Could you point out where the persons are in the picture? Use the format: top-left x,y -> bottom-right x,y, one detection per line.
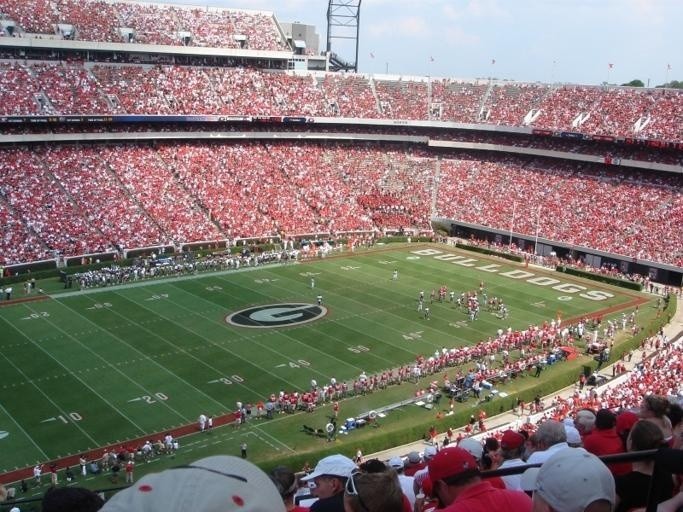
2,49 -> 682,275
2,0 -> 292,53
1,389 -> 300,512
393,269 -> 397,279
5,273 -> 154,299
299,276 -> 683,512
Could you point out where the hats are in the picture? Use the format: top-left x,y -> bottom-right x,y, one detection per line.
103,396 -> 682,510
